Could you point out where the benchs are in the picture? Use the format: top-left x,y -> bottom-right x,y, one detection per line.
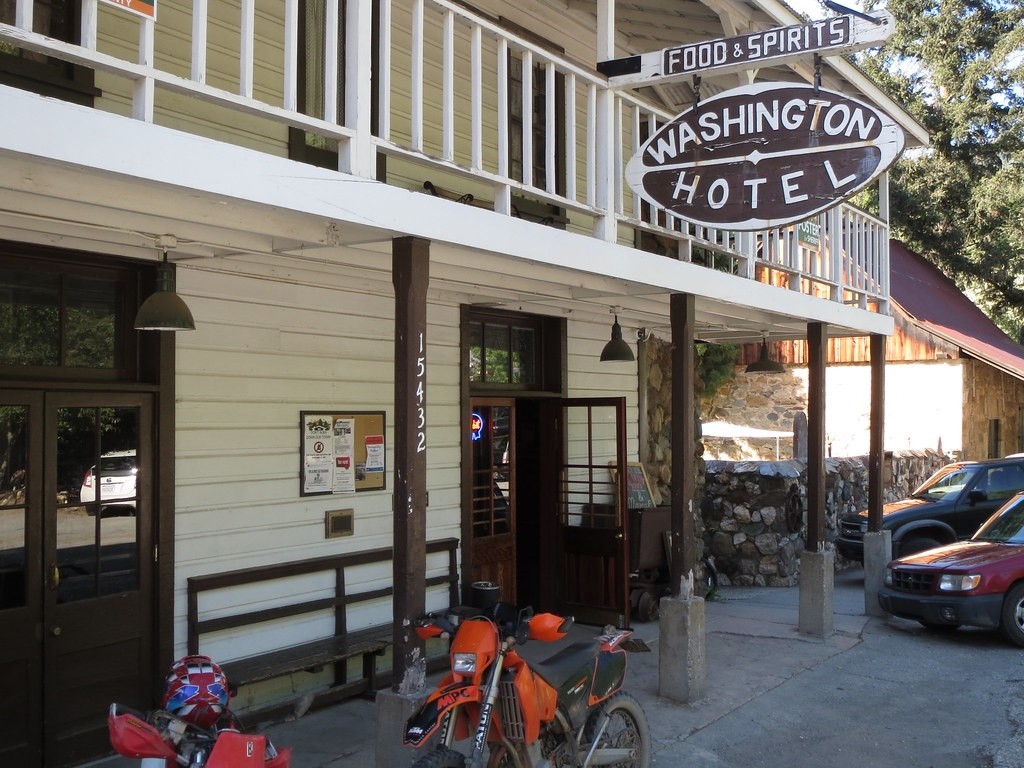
187,536 -> 481,732
423,181 -> 554,226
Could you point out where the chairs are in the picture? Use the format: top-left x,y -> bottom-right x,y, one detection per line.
986,471 -> 1024,499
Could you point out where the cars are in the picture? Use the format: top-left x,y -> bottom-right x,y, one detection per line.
79,449 -> 137,515
876,489 -> 1024,645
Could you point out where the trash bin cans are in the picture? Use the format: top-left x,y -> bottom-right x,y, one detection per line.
471,580 -> 500,609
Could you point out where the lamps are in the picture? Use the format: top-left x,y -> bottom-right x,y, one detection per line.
134,234 -> 196,330
600,306 -> 635,363
745,331 -> 786,373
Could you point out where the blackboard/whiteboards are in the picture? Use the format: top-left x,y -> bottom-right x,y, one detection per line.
608,461 -> 656,508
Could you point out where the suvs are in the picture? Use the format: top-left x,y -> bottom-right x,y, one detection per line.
833,453 -> 1024,574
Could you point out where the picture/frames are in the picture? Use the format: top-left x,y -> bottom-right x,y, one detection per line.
607,460 -> 656,509
299,410 -> 387,498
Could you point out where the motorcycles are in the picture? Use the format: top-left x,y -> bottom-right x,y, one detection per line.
104,653 -> 292,768
402,602 -> 652,768
